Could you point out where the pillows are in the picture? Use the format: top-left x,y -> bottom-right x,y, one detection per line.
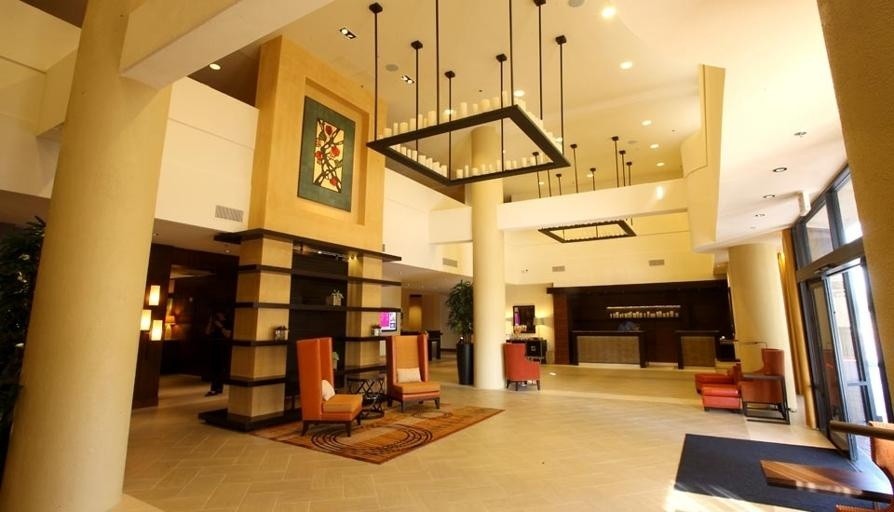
397,368 -> 422,383
321,380 -> 335,401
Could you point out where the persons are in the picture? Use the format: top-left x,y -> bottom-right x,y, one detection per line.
204,305 -> 233,397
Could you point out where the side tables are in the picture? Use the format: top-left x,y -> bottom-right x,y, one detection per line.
347,374 -> 385,419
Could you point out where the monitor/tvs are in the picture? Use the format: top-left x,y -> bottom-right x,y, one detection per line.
380,312 -> 397,332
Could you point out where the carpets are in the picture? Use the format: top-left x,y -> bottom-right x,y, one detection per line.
675,433 -> 890,512
248,386 -> 505,465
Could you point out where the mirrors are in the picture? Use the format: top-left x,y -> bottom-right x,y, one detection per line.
512,306 -> 536,333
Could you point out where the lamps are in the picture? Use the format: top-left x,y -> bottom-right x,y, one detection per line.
532,136 -> 636,243
140,284 -> 163,343
366,0 -> 571,187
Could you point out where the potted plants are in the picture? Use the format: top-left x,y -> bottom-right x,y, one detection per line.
445,280 -> 473,385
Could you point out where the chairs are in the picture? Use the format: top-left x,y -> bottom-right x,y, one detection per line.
386,334 -> 440,413
296,337 -> 362,436
503,344 -> 540,391
738,348 -> 790,425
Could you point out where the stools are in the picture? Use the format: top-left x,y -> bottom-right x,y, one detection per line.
695,373 -> 734,393
701,383 -> 743,413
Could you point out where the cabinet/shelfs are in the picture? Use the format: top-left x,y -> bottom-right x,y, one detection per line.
200,228 -> 401,432
507,339 -> 547,364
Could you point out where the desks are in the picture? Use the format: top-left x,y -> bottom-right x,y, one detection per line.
402,330 -> 443,361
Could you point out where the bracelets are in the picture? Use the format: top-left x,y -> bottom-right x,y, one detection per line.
220,326 -> 224,329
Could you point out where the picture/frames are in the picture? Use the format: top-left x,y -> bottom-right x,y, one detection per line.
298,96 -> 355,213
